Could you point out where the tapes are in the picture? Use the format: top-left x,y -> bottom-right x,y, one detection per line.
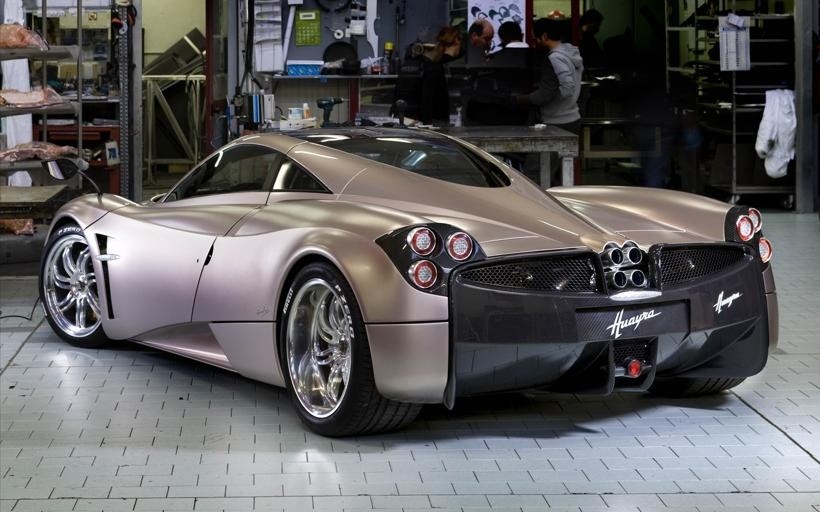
334,29 -> 344,40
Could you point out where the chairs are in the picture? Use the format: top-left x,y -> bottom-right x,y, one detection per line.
272,162 -> 327,190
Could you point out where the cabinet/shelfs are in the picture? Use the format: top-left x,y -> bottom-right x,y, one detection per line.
1,0 -> 83,276
664,0 -> 815,214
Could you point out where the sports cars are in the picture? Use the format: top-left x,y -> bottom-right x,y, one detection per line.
39,124 -> 781,438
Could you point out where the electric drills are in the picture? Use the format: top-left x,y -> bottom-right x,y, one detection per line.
316,96 -> 350,128
392,99 -> 408,129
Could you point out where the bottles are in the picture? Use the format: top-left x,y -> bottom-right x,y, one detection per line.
382,42 -> 398,76
302,103 -> 311,119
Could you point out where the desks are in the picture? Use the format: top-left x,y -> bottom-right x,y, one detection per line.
32,123 -> 122,196
441,124 -> 579,190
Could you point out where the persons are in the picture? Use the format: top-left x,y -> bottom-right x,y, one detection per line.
388,8 -> 605,189
